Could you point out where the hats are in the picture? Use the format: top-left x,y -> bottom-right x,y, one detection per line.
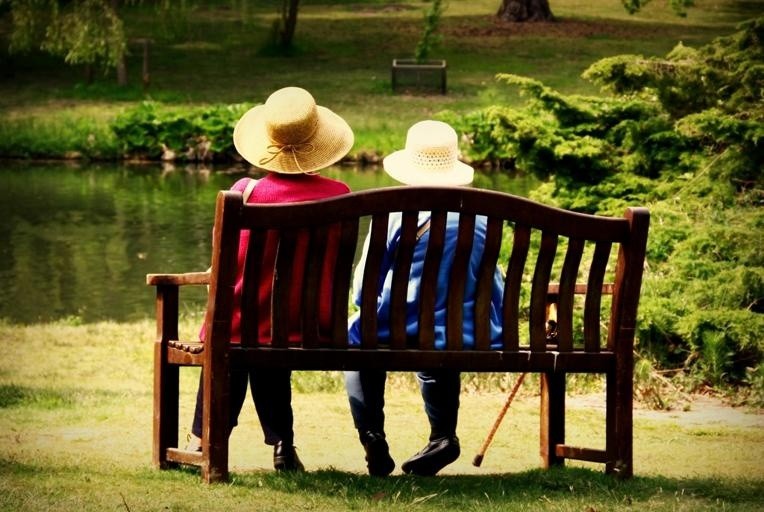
233,86 -> 356,176
382,117 -> 475,188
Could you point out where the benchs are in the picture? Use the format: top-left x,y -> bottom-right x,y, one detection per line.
143,187 -> 652,487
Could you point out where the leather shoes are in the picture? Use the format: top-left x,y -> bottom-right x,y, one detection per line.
401,437 -> 460,477
273,440 -> 305,473
362,431 -> 395,479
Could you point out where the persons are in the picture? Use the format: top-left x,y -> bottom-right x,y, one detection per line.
192,86 -> 358,472
347,119 -> 506,476
